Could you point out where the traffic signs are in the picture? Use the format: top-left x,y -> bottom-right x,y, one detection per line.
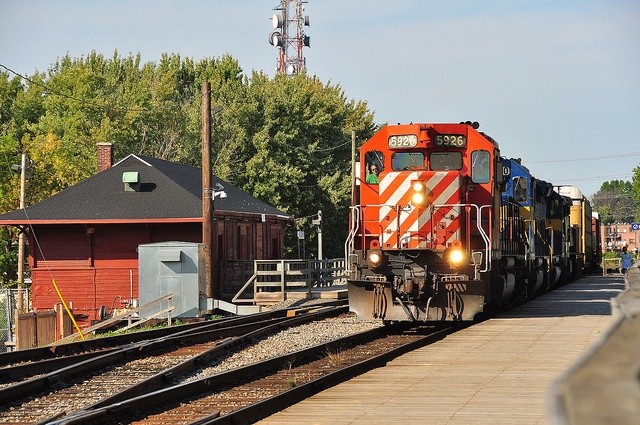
631,222 -> 640,229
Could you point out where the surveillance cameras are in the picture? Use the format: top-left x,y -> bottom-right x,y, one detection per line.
219,191 -> 227,200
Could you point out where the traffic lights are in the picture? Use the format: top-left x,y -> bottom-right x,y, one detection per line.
303,16 -> 310,48
268,11 -> 284,47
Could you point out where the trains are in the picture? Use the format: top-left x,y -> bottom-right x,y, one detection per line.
346,122 -> 602,324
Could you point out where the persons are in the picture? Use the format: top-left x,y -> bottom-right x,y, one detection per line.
620,247 -> 633,274
366,163 -> 380,184
